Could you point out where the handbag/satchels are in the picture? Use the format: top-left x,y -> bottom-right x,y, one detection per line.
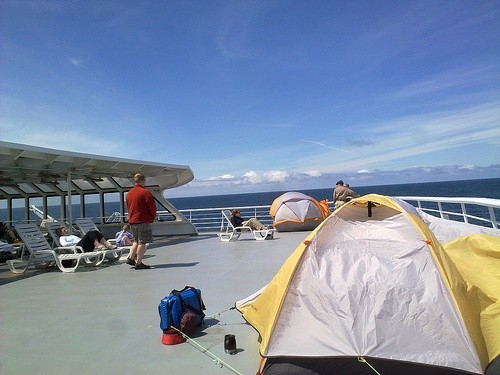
158,286 -> 206,329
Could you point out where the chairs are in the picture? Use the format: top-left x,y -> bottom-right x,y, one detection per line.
5,217 -> 149,273
218,210 -> 275,242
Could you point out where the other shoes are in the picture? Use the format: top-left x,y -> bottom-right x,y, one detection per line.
107,245 -> 117,249
125,257 -> 136,265
135,262 -> 150,269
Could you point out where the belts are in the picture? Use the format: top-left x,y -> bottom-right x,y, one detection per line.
337,200 -> 344,201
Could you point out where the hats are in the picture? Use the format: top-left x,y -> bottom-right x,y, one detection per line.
336,181 -> 343,185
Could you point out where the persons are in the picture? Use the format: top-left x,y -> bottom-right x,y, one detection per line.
56,226 -> 117,253
231,210 -> 267,232
125,173 -> 157,270
332,180 -> 358,209
115,225 -> 133,247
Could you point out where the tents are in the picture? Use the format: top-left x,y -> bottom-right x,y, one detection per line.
233,193 -> 500,375
269,192 -> 331,232
